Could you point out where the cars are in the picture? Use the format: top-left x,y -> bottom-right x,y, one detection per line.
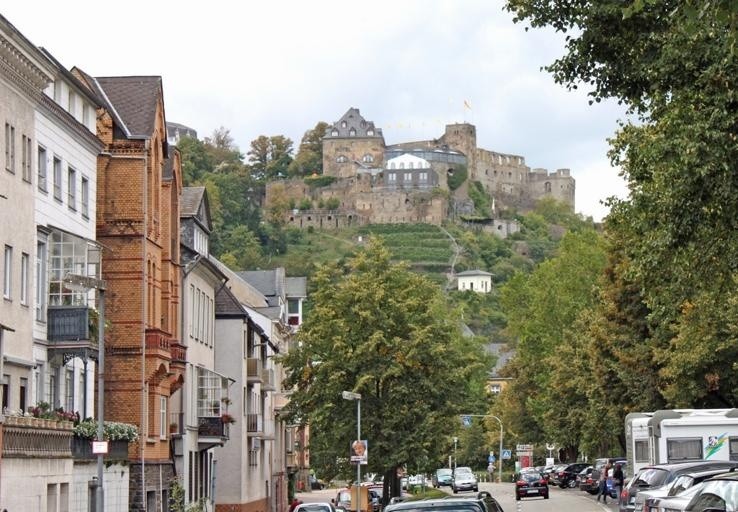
285,466 -> 503,512
511,458 -> 738,512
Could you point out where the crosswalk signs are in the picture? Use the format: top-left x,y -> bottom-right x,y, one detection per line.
501,450 -> 511,460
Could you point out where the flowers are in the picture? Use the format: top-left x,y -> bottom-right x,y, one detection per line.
73,421 -> 139,443
35,401 -> 80,420
88,308 -> 110,330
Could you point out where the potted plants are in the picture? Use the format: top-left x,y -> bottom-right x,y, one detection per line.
222,414 -> 237,425
170,423 -> 177,433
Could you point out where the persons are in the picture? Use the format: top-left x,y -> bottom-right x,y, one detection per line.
289,498 -> 299,512
597,459 -> 624,504
487,462 -> 497,482
351,440 -> 367,461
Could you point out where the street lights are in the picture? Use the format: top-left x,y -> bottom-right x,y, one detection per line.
341,390 -> 363,512
452,435 -> 458,469
61,271 -> 107,511
